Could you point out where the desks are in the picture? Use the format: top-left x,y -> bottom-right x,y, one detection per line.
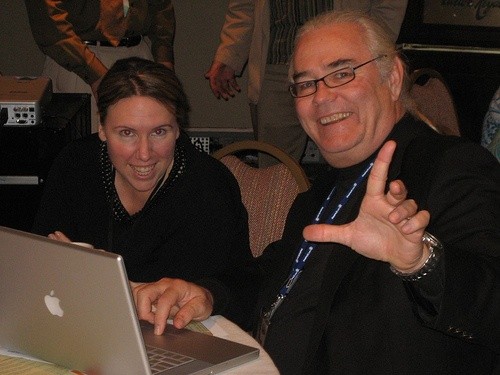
0,283 -> 279,375
0,94 -> 94,176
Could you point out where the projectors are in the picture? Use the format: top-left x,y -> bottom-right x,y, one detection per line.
0,73 -> 54,128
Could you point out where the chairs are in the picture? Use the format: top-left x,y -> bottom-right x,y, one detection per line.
210,139 -> 311,257
409,68 -> 461,138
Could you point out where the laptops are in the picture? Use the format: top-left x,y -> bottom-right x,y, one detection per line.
0,227 -> 259,375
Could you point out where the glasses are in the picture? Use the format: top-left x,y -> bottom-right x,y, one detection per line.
287,54 -> 389,99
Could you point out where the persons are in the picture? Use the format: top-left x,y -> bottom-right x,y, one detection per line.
132,8 -> 500,375
26,0 -> 176,135
31,57 -> 254,283
202,0 -> 408,169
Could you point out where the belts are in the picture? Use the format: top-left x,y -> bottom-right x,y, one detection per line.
82,33 -> 141,48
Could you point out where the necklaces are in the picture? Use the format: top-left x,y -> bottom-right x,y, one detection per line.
99,140 -> 186,224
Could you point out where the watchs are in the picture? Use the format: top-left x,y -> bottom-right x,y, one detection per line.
389,231 -> 445,282
